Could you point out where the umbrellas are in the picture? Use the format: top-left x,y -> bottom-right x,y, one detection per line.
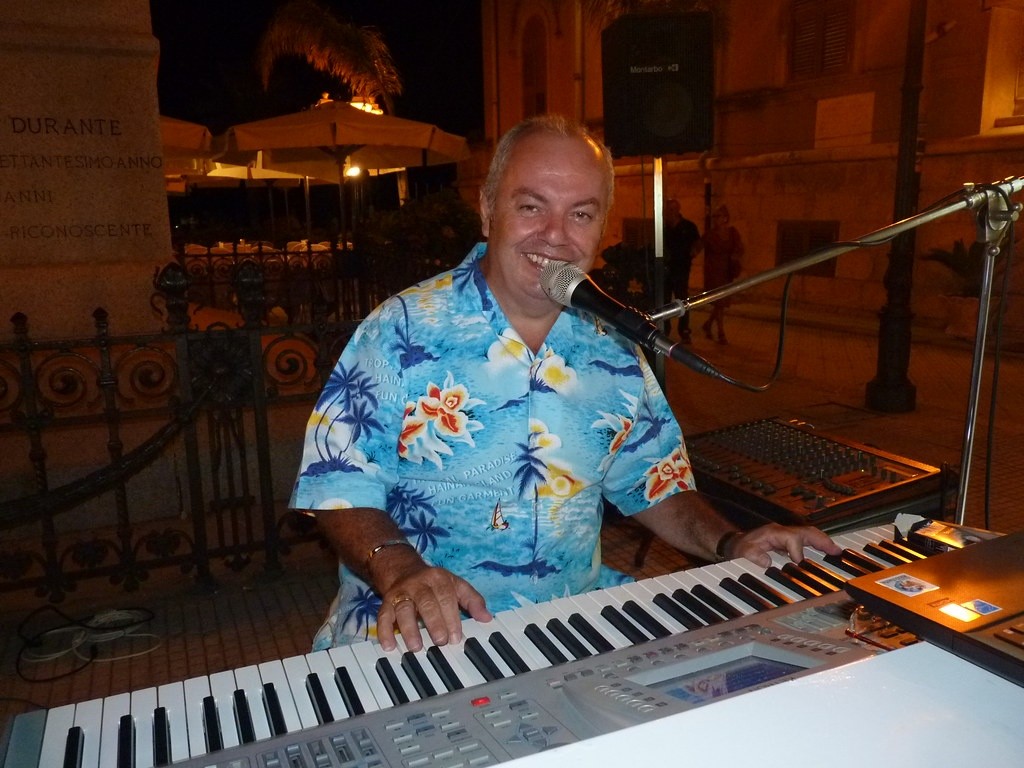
161,100 -> 468,249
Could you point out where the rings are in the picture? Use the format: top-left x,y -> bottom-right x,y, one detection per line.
392,595 -> 419,617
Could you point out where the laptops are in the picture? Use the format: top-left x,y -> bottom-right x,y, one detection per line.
845,530 -> 1024,688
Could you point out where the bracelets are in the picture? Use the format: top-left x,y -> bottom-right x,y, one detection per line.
363,538 -> 417,583
715,530 -> 746,563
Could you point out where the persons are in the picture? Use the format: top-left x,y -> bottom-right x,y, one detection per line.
653,200 -> 703,344
702,206 -> 744,345
288,115 -> 843,652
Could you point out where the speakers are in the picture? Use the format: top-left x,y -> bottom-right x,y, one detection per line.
602,10 -> 714,158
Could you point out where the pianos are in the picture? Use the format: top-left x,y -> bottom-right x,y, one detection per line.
1,512 -> 1024,768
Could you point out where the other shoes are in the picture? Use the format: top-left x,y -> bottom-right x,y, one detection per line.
718,333 -> 728,344
703,322 -> 713,339
680,329 -> 692,343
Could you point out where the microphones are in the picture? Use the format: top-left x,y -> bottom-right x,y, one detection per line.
539,258 -> 721,377
